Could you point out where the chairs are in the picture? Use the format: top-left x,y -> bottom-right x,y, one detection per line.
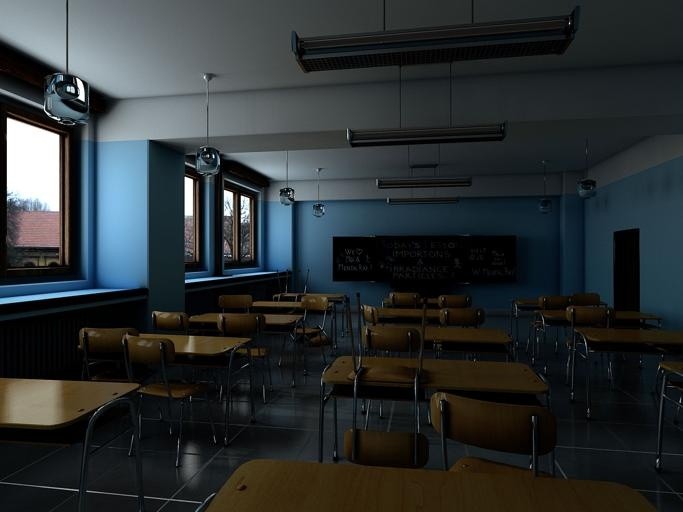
277,269 -> 310,313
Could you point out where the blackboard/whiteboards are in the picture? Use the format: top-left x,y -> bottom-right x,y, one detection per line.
331,233 -> 521,285
